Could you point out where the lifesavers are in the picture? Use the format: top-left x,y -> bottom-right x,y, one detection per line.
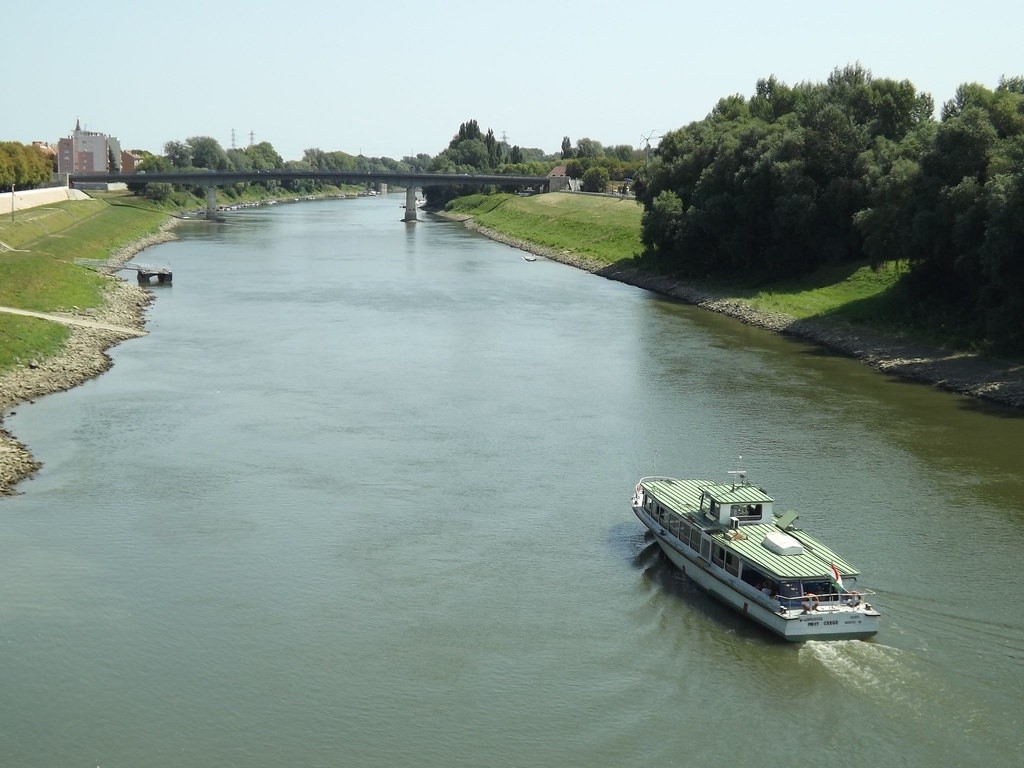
846,590 -> 862,607
801,593 -> 818,611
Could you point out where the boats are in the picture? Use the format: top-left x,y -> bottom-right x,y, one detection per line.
629,456 -> 883,640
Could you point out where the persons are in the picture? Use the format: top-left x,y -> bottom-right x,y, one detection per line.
754,580 -> 772,596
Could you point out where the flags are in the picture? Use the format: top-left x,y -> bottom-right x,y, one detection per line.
831,562 -> 843,587
